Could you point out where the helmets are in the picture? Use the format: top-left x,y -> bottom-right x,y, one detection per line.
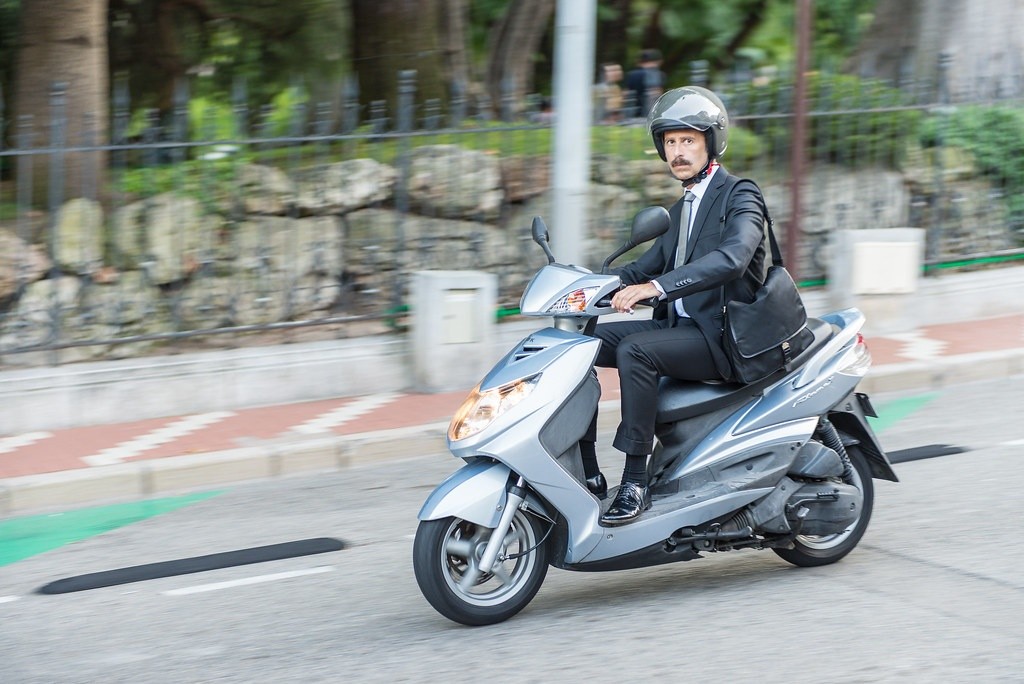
645,86 -> 729,163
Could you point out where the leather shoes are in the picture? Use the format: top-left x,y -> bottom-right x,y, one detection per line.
602,481 -> 653,526
586,474 -> 607,500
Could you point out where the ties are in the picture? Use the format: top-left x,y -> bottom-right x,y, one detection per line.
667,192 -> 696,326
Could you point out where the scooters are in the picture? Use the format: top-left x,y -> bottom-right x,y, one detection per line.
411,203 -> 901,627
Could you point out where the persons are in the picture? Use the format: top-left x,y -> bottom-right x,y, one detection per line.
580,86 -> 767,523
530,46 -> 777,129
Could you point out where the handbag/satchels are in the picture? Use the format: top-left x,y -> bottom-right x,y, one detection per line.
722,266 -> 816,386
